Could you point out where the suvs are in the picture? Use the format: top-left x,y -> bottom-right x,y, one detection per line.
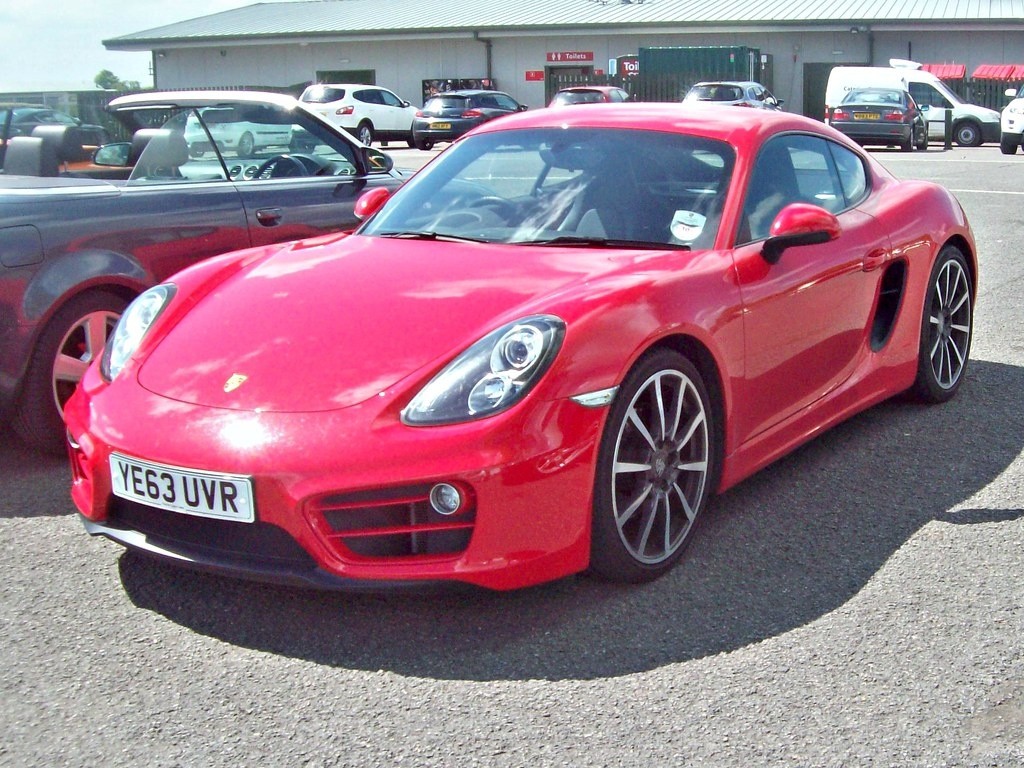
291,83 -> 421,154
184,106 -> 291,158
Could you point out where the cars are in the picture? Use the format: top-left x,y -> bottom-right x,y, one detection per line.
681,80 -> 784,111
1000,82 -> 1024,155
540,85 -> 636,143
0,108 -> 182,178
412,89 -> 527,150
830,85 -> 929,153
64,102 -> 979,590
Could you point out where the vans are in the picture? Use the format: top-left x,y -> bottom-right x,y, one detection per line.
825,59 -> 1002,146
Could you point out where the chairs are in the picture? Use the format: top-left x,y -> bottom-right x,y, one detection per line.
557,166 -> 640,241
691,181 -> 784,244
31,125 -> 89,178
130,129 -> 189,180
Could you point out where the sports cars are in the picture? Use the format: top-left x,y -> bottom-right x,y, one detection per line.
0,92 -> 493,459
